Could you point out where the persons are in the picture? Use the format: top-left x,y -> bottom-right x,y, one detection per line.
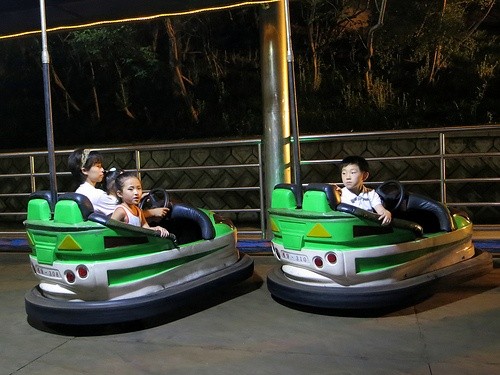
68,148 -> 169,237
333,155 -> 392,225
